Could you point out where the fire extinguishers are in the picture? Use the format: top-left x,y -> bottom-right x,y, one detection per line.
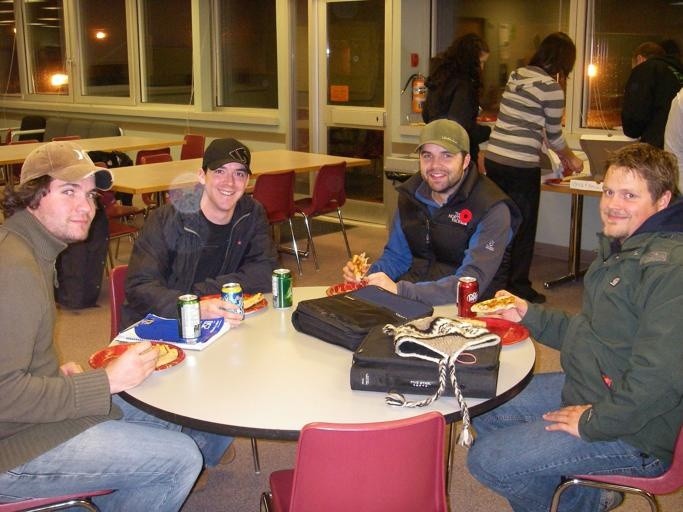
401,73 -> 427,113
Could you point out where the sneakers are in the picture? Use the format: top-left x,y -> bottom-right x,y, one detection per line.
507,284 -> 546,304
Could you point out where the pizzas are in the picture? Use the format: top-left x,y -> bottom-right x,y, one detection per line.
244,293 -> 264,309
352,252 -> 371,281
143,343 -> 169,356
471,295 -> 516,313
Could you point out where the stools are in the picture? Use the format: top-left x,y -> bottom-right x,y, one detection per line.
0,479 -> 114,512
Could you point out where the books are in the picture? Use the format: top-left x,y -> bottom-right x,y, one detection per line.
115,314 -> 230,351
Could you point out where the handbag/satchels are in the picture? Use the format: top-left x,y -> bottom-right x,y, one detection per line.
351,324 -> 501,398
291,285 -> 433,351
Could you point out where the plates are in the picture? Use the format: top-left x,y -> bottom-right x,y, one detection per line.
470,316 -> 530,346
326,279 -> 366,296
199,293 -> 268,316
87,340 -> 186,374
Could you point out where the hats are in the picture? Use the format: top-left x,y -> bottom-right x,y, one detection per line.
202,139 -> 252,176
414,119 -> 471,156
20,140 -> 114,191
382,315 -> 501,448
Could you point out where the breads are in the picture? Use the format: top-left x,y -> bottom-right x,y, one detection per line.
156,348 -> 179,368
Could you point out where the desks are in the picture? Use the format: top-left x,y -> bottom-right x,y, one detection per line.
540,167 -> 609,291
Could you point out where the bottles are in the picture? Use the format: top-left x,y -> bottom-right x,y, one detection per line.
177,294 -> 201,339
221,282 -> 245,324
271,268 -> 293,310
456,277 -> 480,319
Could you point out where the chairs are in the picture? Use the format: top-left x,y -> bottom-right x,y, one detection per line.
179,133 -> 206,160
40,118 -> 123,144
253,171 -> 302,276
3,116 -> 44,145
109,265 -> 128,349
550,416 -> 683,511
94,162 -> 135,229
141,154 -> 179,221
104,220 -> 137,275
262,413 -> 447,512
293,161 -> 351,265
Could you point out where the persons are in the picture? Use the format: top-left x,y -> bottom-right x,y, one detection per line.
484,31 -> 583,303
113,137 -> 279,491
422,33 -> 490,176
343,120 -> 521,308
662,85 -> 683,197
467,142 -> 682,511
0,142 -> 236,512
620,40 -> 683,148
